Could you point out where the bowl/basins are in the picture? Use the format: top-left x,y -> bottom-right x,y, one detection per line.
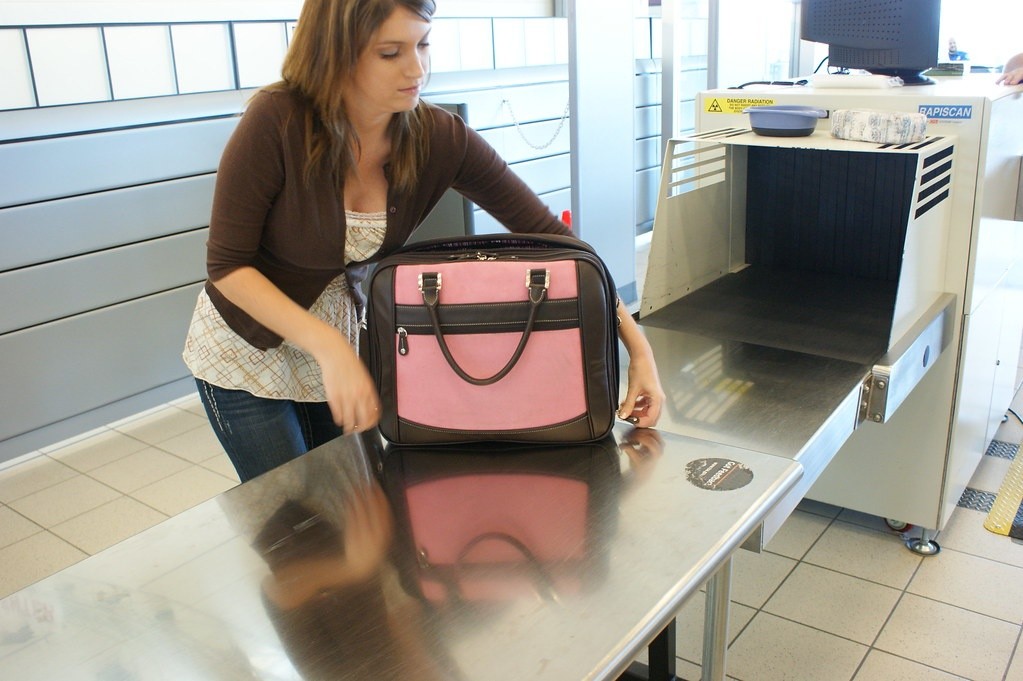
741,105 -> 827,137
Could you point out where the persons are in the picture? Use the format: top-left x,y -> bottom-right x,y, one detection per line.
220,427 -> 665,680
182,2 -> 667,482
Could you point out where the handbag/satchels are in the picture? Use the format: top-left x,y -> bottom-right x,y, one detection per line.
376,444 -> 617,621
365,231 -> 621,448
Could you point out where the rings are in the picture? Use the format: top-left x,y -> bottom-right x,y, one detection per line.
373,406 -> 383,411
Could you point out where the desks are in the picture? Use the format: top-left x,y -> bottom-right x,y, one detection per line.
0,426 -> 806,680
